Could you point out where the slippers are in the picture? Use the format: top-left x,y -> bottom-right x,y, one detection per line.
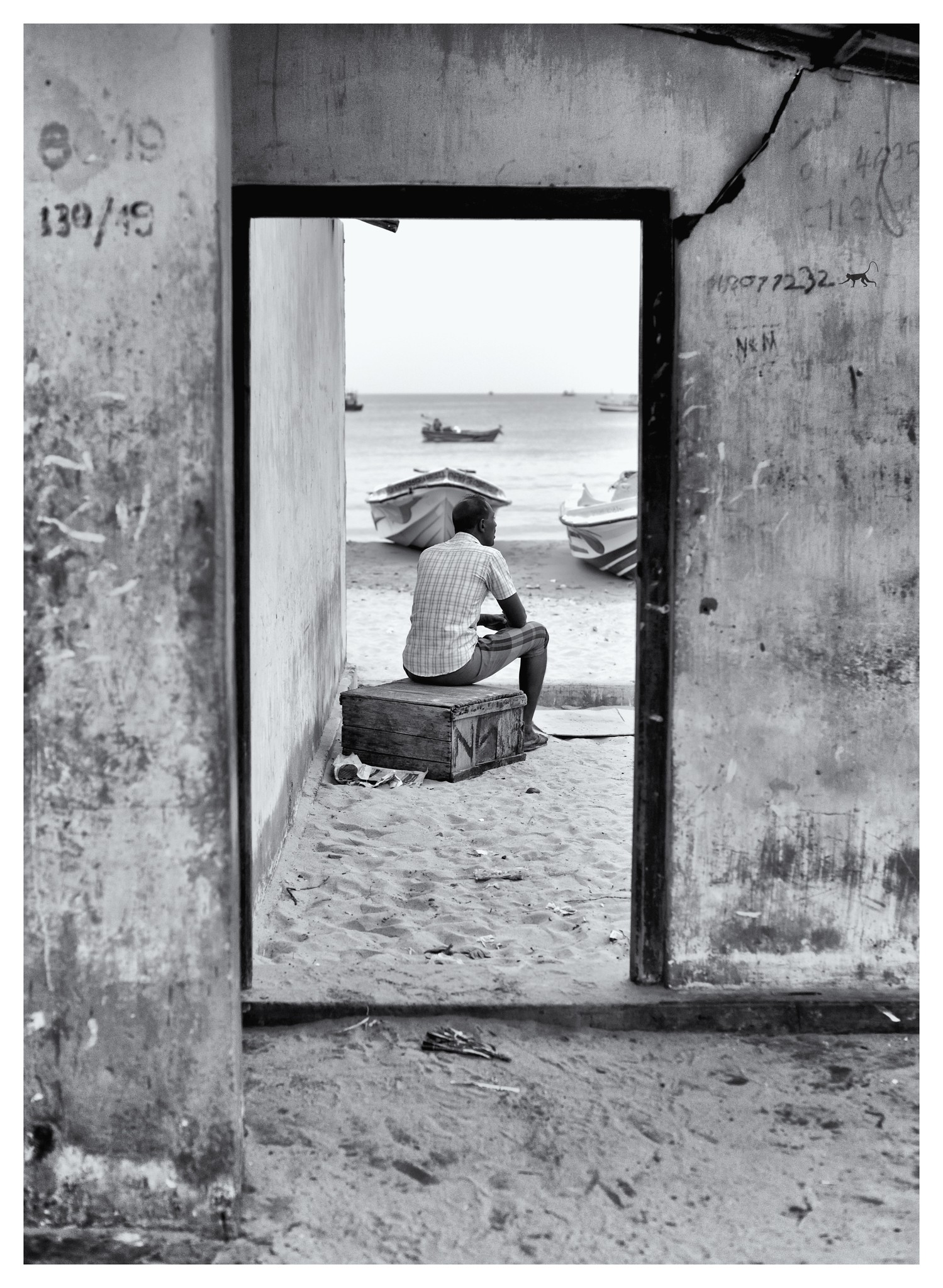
524,733 -> 548,751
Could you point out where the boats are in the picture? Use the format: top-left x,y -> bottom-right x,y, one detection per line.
557,473 -> 637,584
364,466 -> 511,555
422,418 -> 502,441
345,392 -> 363,411
595,399 -> 639,413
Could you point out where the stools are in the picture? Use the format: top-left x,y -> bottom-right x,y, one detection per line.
339,679 -> 527,783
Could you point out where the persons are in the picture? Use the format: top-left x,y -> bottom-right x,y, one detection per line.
402,493 -> 550,752
